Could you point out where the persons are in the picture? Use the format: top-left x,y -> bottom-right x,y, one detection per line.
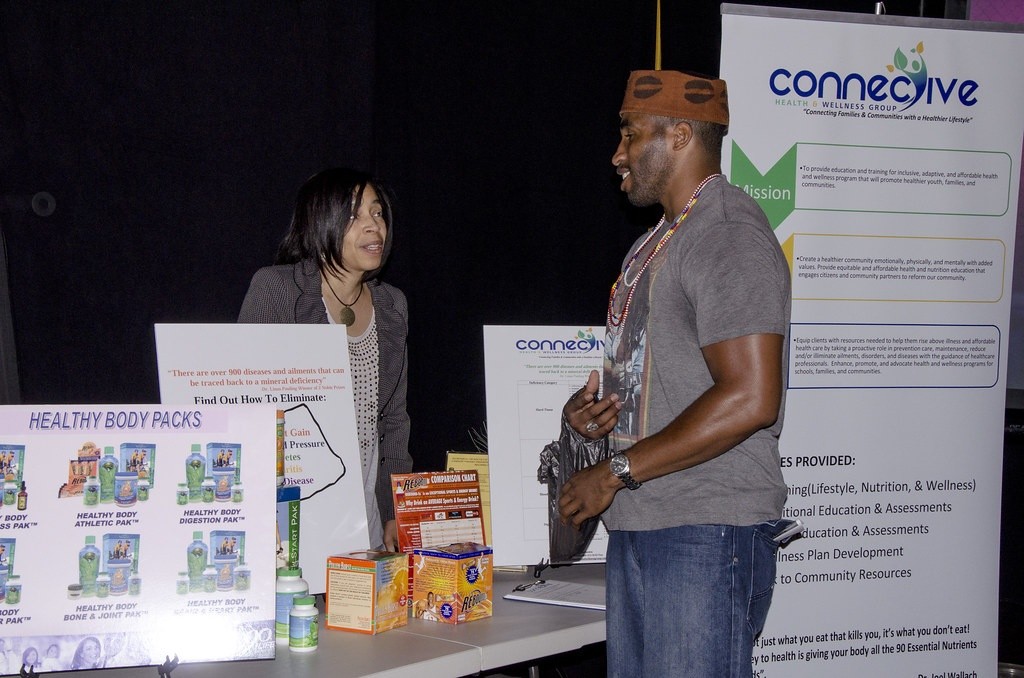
557,68 -> 793,675
234,166 -> 413,553
4,451 -> 16,475
136,450 -> 146,472
226,537 -> 238,555
220,537 -> 229,555
0,451 -> 6,474
113,541 -> 123,558
223,450 -> 233,468
416,592 -> 445,623
217,449 -> 225,468
130,449 -> 139,471
120,541 -> 132,560
0,544 -> 6,566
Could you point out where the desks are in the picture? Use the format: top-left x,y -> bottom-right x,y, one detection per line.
0,562 -> 610,678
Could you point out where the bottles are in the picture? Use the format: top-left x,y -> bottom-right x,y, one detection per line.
83,475 -> 100,505
276,410 -> 286,486
5,575 -> 21,604
3,479 -> 18,505
275,566 -> 309,646
0,473 -> 5,507
289,595 -> 319,652
0,565 -> 8,603
137,477 -> 150,501
175,531 -> 251,595
98,446 -> 119,504
67,535 -> 142,598
114,472 -> 138,506
18,481 -> 28,510
230,482 -> 244,503
212,467 -> 235,502
176,483 -> 189,505
201,476 -> 216,502
185,443 -> 206,503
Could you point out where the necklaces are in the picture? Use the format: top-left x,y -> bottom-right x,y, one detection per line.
607,172 -> 727,338
321,267 -> 364,327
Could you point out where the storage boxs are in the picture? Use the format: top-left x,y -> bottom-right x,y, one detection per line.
413,541 -> 493,624
0,538 -> 16,575
210,530 -> 245,566
0,444 -> 25,491
276,484 -> 301,567
120,442 -> 156,488
325,549 -> 408,636
206,442 -> 241,485
103,533 -> 140,573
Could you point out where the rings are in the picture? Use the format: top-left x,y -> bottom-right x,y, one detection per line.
587,420 -> 599,433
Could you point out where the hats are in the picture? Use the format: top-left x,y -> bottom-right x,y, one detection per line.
618,70 -> 730,126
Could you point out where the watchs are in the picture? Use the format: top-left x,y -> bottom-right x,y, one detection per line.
610,448 -> 643,491
